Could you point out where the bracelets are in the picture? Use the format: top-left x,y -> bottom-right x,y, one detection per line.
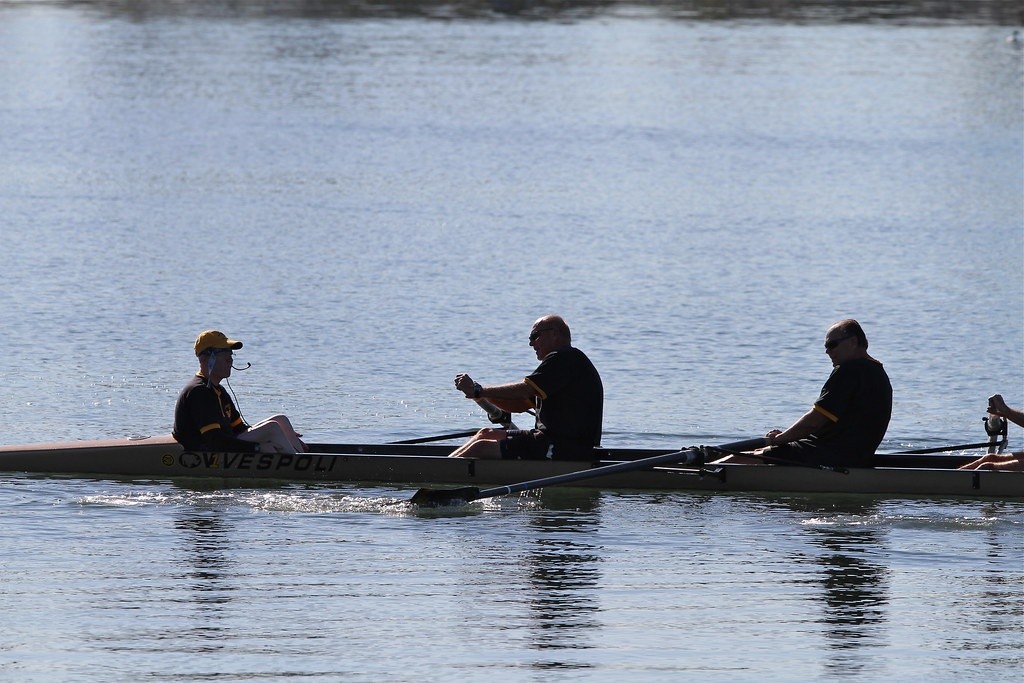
255,443 -> 260,452
999,405 -> 1007,411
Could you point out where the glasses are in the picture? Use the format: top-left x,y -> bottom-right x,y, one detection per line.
825,335 -> 855,350
529,328 -> 555,340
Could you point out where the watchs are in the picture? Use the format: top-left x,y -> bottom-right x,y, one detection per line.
473,383 -> 482,397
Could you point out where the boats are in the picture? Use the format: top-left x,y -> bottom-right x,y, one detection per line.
1,435 -> 1024,502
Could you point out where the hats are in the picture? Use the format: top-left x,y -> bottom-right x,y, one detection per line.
195,330 -> 242,357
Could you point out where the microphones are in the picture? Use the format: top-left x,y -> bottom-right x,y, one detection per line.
232,362 -> 251,370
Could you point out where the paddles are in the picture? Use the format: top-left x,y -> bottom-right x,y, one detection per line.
984,409 -> 1005,452
408,437 -> 768,510
466,394 -> 520,433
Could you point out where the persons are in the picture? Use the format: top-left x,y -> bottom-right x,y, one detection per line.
958,395 -> 1024,470
449,315 -> 605,460
173,330 -> 304,453
710,320 -> 893,469
